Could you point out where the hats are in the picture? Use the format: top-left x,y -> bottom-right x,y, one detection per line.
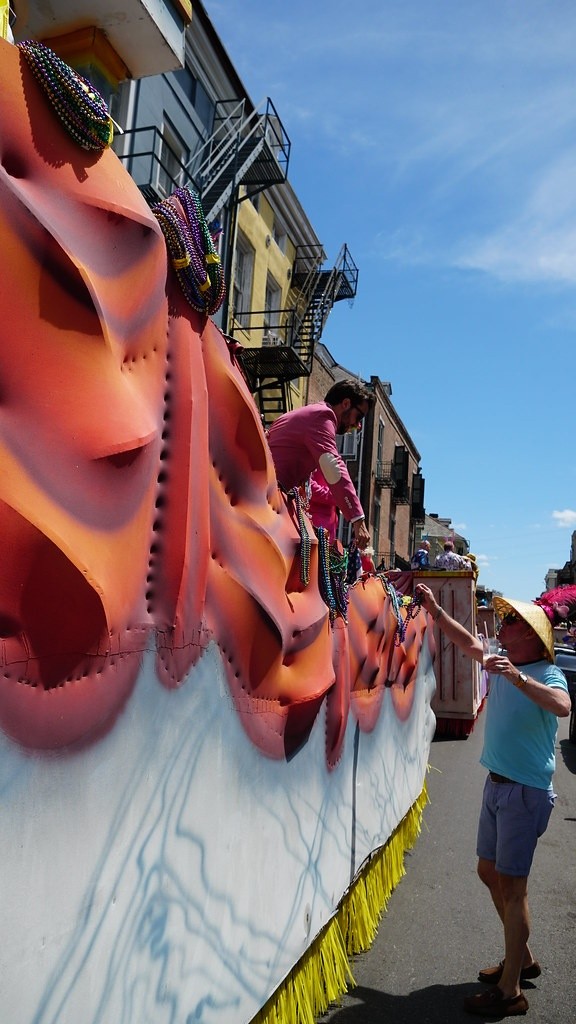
492,584 -> 576,664
462,553 -> 476,565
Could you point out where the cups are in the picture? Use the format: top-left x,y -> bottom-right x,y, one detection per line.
482,638 -> 499,670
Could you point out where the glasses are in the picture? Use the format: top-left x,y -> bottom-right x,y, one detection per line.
353,405 -> 364,422
502,614 -> 528,625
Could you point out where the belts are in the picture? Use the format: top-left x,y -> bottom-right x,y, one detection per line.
489,771 -> 517,784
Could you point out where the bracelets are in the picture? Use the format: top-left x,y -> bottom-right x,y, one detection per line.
434,606 -> 443,623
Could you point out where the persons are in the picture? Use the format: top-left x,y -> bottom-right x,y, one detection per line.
266,377 -> 378,551
411,540 -> 431,565
433,541 -> 471,572
415,583 -> 572,1016
306,467 -> 340,543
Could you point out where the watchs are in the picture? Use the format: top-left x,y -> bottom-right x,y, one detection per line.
513,671 -> 528,688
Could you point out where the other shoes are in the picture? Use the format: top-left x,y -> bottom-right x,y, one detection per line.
463,989 -> 530,1014
479,959 -> 541,979
